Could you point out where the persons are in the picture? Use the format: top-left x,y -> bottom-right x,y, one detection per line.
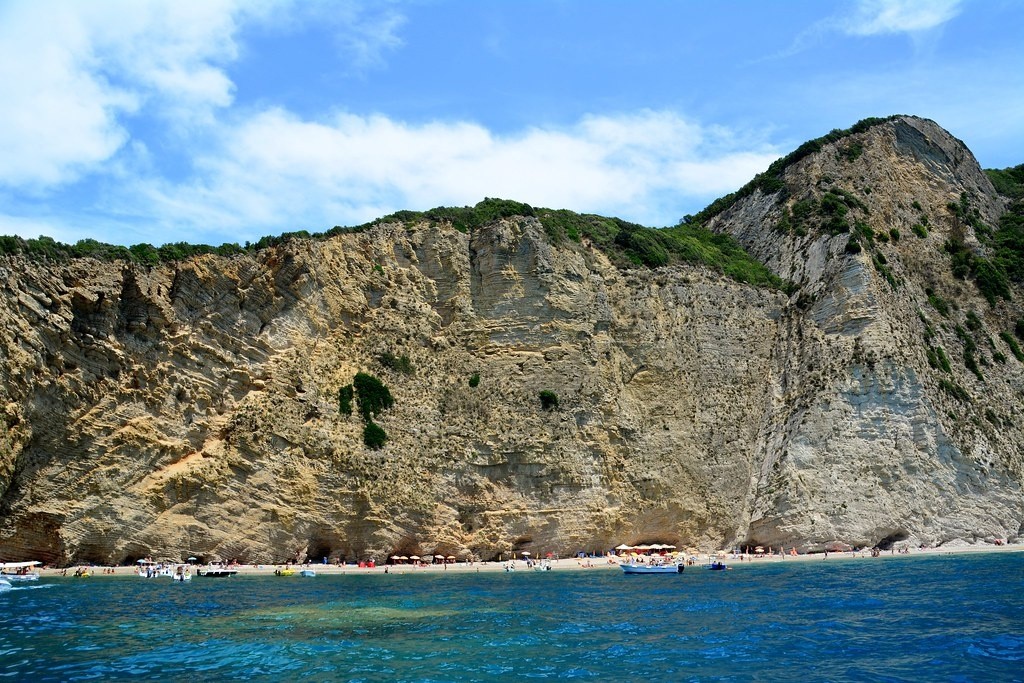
323,556 -> 380,568
0,562 -> 119,577
466,555 -> 750,568
769,541 -> 930,560
993,538 -> 1009,544
136,559 -> 242,573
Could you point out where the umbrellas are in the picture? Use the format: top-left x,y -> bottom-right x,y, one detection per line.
138,559 -> 146,568
188,557 -> 197,564
391,555 -> 455,564
755,545 -> 763,555
615,542 -> 677,555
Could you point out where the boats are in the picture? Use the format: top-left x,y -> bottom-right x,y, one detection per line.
301,570 -> 316,577
196,559 -> 238,577
172,564 -> 193,581
709,554 -> 726,571
614,543 -> 679,573
1,560 -> 42,581
139,558 -> 158,579
274,570 -> 295,578
